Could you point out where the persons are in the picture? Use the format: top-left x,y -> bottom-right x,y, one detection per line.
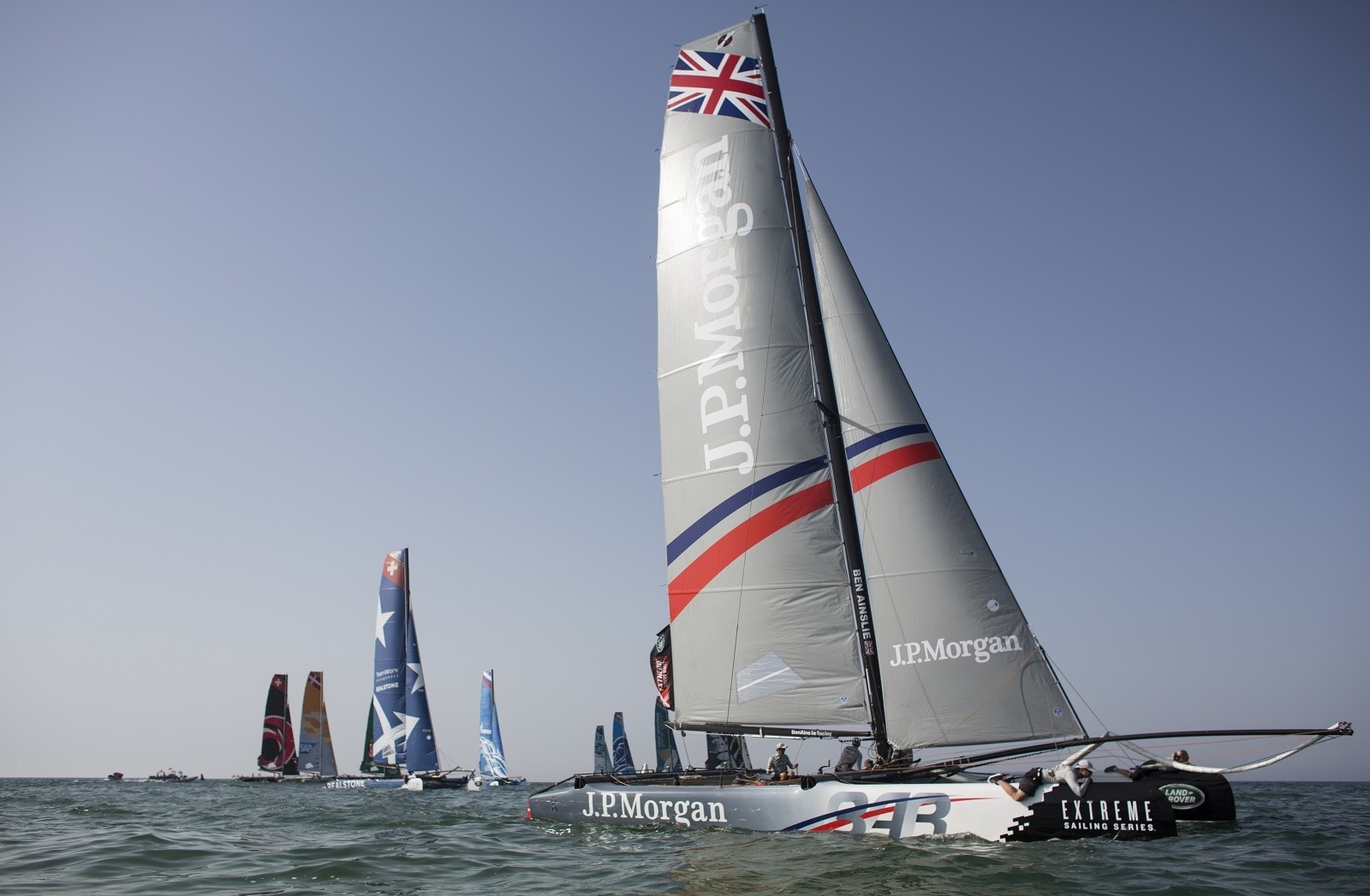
1104,749 -> 1190,779
835,738 -> 904,772
766,743 -> 798,781
987,760 -> 1097,802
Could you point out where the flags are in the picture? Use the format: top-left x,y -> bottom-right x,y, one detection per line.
650,624 -> 675,711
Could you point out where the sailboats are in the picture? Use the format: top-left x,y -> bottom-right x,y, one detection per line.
521,3 -> 1354,841
479,668 -> 527,786
234,546 -> 483,792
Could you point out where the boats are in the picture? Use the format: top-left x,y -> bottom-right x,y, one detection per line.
147,771 -> 199,783
108,772 -> 124,781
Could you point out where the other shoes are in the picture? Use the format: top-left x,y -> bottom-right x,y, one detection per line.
1103,766 -> 1116,773
988,774 -> 1002,785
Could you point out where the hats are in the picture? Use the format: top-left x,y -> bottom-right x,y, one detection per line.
776,742 -> 789,750
853,738 -> 860,746
1074,760 -> 1095,772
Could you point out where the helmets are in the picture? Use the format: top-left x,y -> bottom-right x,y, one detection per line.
1173,750 -> 1188,764
